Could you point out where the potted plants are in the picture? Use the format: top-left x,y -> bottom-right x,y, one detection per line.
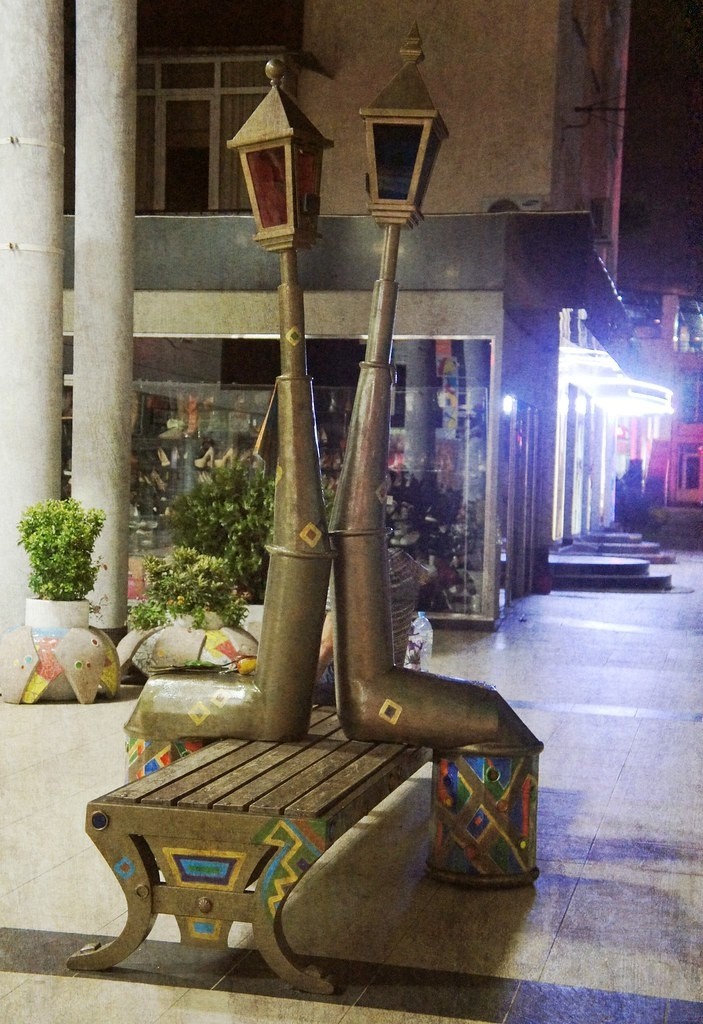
17,498 -> 106,628
123,547 -> 252,633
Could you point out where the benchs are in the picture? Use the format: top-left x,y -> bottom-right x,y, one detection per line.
66,702 -> 432,994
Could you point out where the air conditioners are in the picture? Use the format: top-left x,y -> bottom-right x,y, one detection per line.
482,193 -> 543,213
591,197 -> 613,249
